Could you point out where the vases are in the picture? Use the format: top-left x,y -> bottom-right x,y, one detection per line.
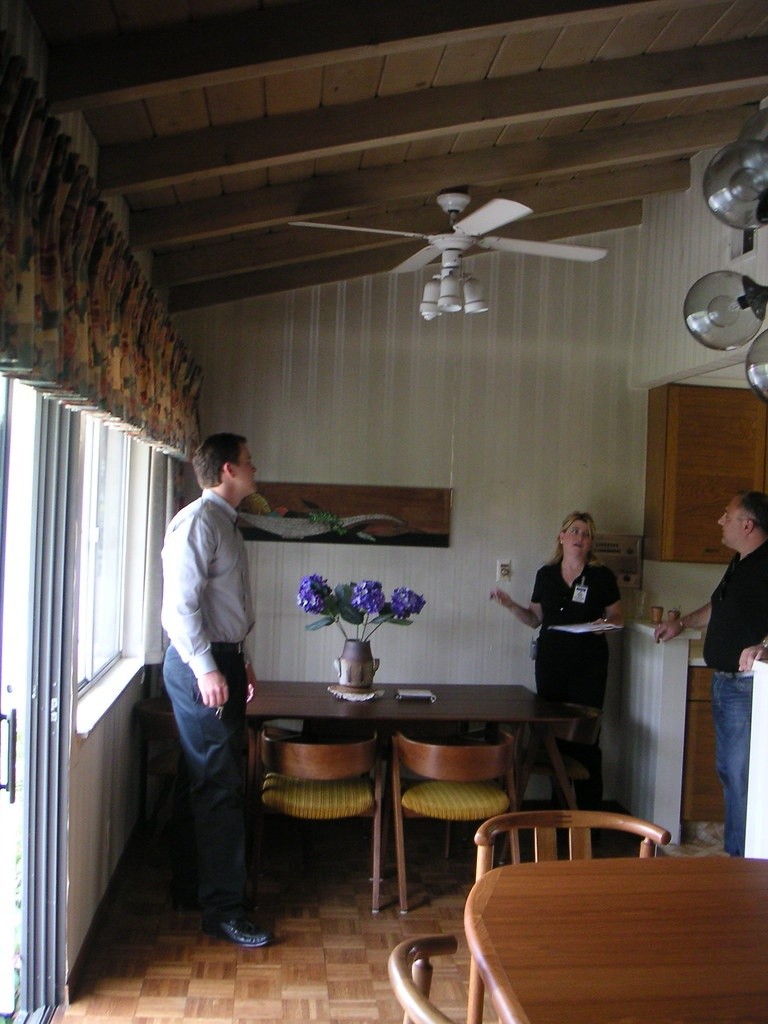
335,639 -> 379,687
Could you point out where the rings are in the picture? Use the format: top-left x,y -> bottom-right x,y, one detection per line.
658,623 -> 660,628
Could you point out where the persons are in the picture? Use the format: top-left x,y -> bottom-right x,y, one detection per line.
489,511 -> 623,858
164,432 -> 275,948
654,490 -> 768,859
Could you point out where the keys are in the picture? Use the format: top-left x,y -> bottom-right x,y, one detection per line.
215,704 -> 223,719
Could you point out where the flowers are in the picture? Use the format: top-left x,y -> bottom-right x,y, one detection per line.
296,575 -> 426,643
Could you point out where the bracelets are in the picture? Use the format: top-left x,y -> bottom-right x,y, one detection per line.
679,617 -> 684,628
760,638 -> 768,647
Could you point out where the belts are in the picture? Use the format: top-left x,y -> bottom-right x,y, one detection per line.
715,672 -> 746,680
211,641 -> 243,655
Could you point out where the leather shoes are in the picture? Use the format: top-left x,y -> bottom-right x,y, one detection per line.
201,909 -> 274,947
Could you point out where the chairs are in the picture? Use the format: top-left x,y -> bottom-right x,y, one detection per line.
388,936 -> 473,1023
133,700 -> 607,917
468,810 -> 672,1024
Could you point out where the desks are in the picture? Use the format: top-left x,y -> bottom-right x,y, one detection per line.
237,679 -> 587,856
463,841 -> 768,1023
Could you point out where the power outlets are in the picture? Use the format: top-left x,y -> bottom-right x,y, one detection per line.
618,573 -> 641,589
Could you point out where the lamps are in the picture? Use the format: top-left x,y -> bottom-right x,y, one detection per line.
418,270 -> 488,319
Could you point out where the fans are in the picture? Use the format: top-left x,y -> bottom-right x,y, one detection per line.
282,191 -> 609,275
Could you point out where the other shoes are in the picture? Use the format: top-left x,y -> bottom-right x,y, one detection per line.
170,887 -> 257,912
